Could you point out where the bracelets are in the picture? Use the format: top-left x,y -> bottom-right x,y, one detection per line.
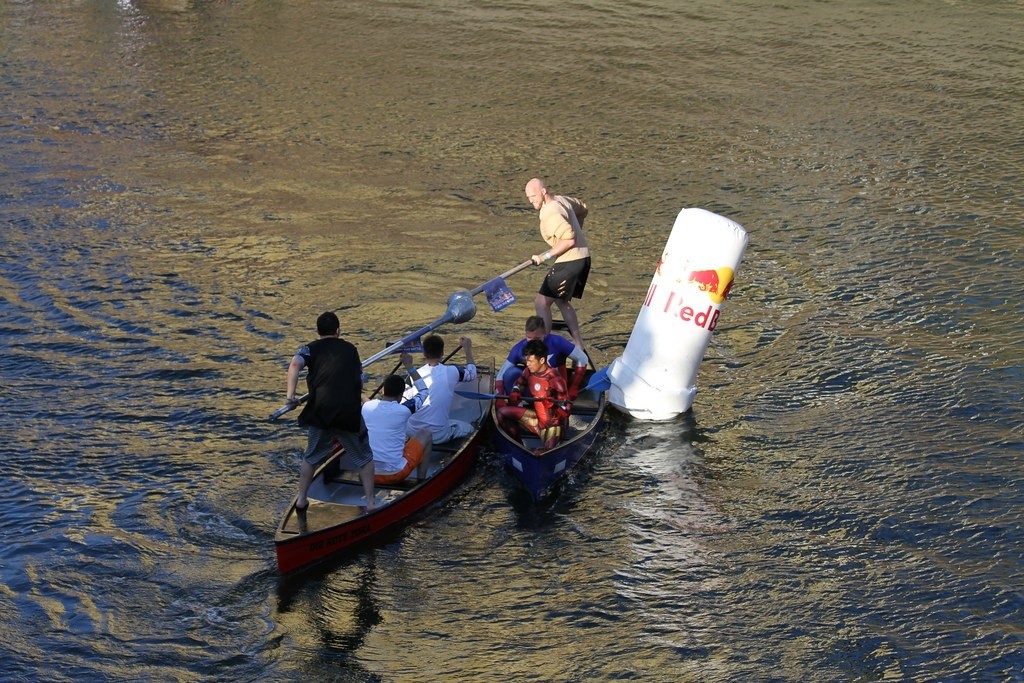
546,253 -> 552,260
286,399 -> 294,400
408,367 -> 416,374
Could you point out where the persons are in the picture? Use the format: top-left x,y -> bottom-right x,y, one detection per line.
525,177 -> 591,369
409,335 -> 478,444
497,316 -> 588,448
361,352 -> 432,486
285,311 -> 386,512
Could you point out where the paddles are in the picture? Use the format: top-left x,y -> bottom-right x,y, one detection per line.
441,343 -> 467,366
454,389 -> 570,407
570,364 -> 612,400
365,357 -> 406,402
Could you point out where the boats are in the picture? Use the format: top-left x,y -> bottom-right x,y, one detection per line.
491,317 -> 607,505
274,356 -> 494,573
